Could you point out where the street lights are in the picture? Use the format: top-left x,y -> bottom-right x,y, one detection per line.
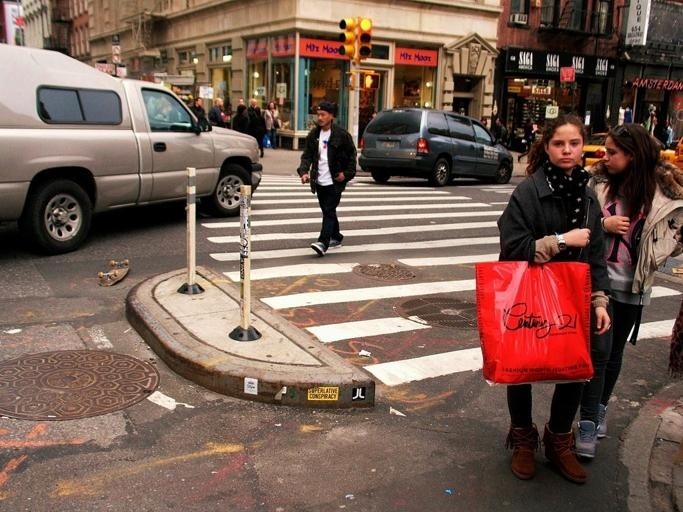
221,49 -> 234,106
251,68 -> 259,101
190,53 -> 199,97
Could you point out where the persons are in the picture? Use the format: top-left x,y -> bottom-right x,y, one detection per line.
572,122 -> 682,462
480,118 -> 538,161
296,101 -> 357,257
617,103 -> 674,149
190,97 -> 278,158
496,115 -> 611,486
582,111 -> 594,128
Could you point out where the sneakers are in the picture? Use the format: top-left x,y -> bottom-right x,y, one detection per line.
328,240 -> 341,248
574,420 -> 600,459
310,242 -> 327,255
597,404 -> 607,438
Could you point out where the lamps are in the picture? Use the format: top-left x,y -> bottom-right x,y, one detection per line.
221,45 -> 244,63
188,51 -> 205,66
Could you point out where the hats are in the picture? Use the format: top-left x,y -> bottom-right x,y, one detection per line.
316,101 -> 336,113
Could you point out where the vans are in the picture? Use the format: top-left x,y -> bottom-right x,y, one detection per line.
0,41 -> 262,254
358,107 -> 514,186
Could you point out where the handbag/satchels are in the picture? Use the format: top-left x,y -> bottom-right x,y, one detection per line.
474,259 -> 593,387
263,130 -> 271,148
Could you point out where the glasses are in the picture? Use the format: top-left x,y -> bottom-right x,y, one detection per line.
610,125 -> 630,137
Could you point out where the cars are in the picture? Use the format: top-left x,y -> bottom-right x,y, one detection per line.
581,131 -> 610,169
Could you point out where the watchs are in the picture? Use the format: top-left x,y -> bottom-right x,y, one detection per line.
556,234 -> 566,254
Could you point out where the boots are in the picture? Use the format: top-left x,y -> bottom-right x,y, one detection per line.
543,424 -> 587,483
505,421 -> 541,480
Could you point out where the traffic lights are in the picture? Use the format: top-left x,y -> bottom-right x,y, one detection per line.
344,71 -> 356,91
360,70 -> 380,90
358,18 -> 373,58
339,17 -> 355,56
